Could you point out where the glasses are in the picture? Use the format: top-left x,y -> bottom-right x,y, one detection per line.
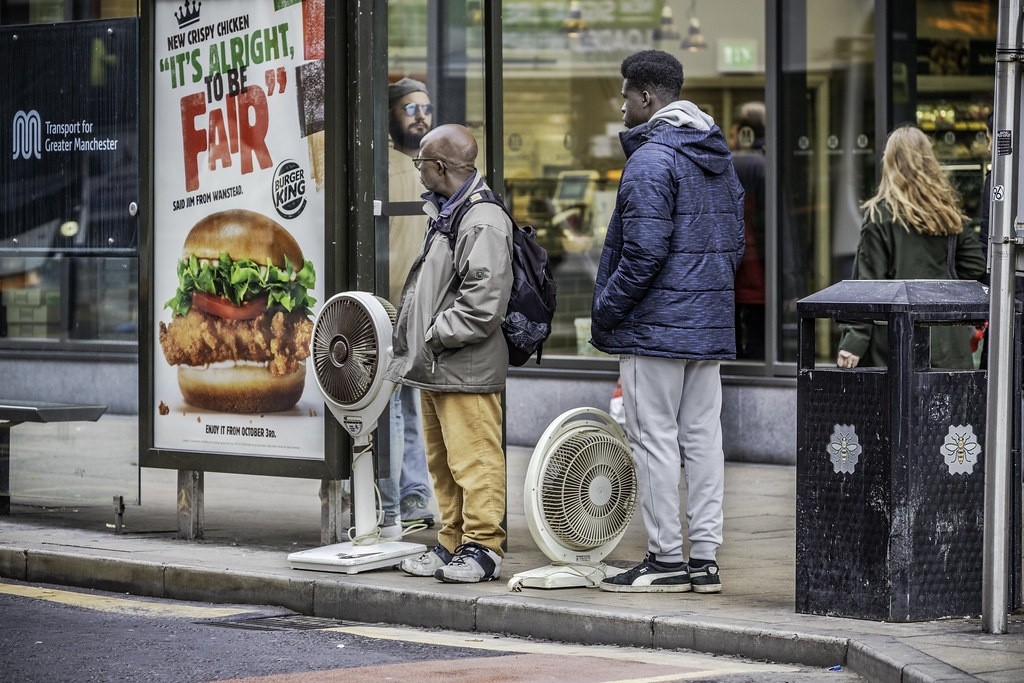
394,104 -> 434,117
412,155 -> 448,170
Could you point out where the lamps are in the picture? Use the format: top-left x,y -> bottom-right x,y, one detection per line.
679,0 -> 707,52
652,0 -> 680,41
562,2 -> 587,37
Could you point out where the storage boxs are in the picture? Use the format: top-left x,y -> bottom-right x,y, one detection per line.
1,284 -> 62,338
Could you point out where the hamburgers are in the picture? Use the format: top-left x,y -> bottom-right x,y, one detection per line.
160,207 -> 316,414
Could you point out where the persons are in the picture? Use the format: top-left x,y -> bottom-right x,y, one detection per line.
724,119 -> 802,363
588,51 -> 744,592
339,78 -> 433,539
838,127 -> 986,370
382,124 -> 514,584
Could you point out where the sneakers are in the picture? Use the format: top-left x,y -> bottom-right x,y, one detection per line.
400,543 -> 454,579
436,543 -> 502,582
599,552 -> 691,594
399,493 -> 436,529
685,561 -> 722,593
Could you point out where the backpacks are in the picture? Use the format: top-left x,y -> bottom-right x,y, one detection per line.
449,190 -> 557,368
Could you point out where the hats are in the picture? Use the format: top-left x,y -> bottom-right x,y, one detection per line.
387,78 -> 429,105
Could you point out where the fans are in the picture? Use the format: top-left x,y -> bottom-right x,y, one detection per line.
508,406 -> 638,593
288,291 -> 427,575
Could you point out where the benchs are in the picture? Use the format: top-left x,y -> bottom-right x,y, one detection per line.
0,402 -> 108,515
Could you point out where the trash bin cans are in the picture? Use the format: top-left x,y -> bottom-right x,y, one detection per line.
796,279 -> 1024,616
